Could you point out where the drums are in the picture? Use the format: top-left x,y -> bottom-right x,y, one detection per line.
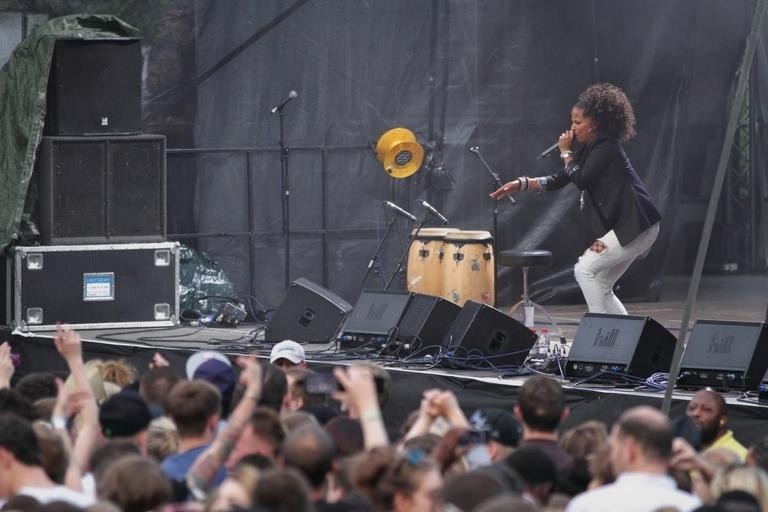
442,230 -> 495,310
407,228 -> 459,300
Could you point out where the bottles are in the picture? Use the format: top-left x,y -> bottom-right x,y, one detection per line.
529,328 -> 538,356
538,328 -> 550,354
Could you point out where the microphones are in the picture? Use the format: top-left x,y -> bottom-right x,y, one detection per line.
384,201 -> 417,224
423,200 -> 449,225
536,133 -> 576,161
270,91 -> 298,114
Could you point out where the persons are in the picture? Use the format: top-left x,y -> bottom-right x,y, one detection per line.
486,82 -> 661,315
1,321 -> 767,511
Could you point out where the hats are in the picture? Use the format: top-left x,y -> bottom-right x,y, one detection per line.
184,348 -> 238,403
468,407 -> 525,449
269,338 -> 307,366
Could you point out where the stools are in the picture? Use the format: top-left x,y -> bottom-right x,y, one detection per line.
495,248 -> 555,340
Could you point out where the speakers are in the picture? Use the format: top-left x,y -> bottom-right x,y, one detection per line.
37,135 -> 168,246
341,290 -> 415,353
390,293 -> 461,357
45,38 -> 144,136
435,299 -> 537,370
265,277 -> 354,344
562,312 -> 677,386
676,319 -> 768,392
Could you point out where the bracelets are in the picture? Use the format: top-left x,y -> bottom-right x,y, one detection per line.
517,176 -> 530,193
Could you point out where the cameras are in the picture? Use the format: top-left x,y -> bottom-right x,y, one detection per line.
306,372 -> 344,395
460,430 -> 489,443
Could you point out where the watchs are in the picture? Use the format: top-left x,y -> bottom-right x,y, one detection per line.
559,149 -> 573,160
